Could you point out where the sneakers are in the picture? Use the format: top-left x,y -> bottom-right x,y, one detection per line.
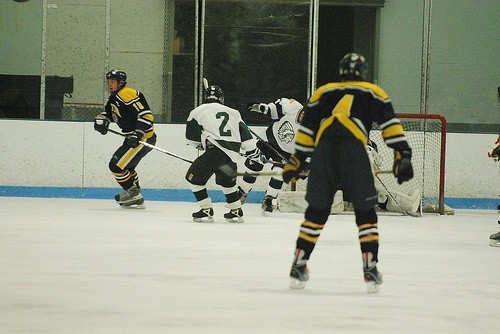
115,181 -> 140,201
192,207 -> 214,222
224,207 -> 245,223
260,190 -> 277,215
288,248 -> 309,289
117,184 -> 146,209
363,264 -> 383,293
225,186 -> 252,208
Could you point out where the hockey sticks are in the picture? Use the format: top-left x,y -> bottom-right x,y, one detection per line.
220,163 -> 393,177
108,128 -> 194,163
202,77 -> 287,161
376,174 -> 419,216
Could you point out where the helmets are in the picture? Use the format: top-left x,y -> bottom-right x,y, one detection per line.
106,70 -> 127,89
339,52 -> 369,81
205,85 -> 225,105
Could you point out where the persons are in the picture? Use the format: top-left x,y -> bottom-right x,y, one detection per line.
185,85 -> 266,222
225,98 -> 311,216
94,69 -> 157,209
283,53 -> 414,293
337,138 -> 420,214
488,86 -> 500,247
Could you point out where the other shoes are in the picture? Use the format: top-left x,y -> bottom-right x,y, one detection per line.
489,231 -> 500,247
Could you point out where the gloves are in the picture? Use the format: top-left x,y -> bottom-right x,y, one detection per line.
94,115 -> 110,135
394,148 -> 414,184
196,144 -> 204,151
281,149 -> 311,184
124,129 -> 145,148
246,103 -> 271,115
246,148 -> 266,166
